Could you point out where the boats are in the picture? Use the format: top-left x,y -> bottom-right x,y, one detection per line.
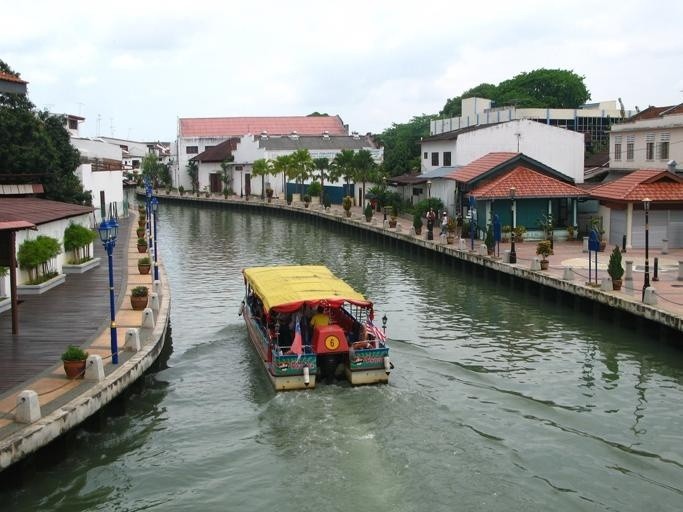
238,264 -> 394,393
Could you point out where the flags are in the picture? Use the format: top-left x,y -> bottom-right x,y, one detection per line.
366,319 -> 386,342
290,316 -> 302,360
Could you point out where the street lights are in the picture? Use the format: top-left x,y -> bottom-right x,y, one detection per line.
425,180 -> 435,241
140,175 -> 160,282
641,196 -> 654,301
95,215 -> 122,365
383,176 -> 388,221
507,186 -> 518,264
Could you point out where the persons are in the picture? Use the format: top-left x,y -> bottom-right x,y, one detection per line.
439,211 -> 451,237
453,212 -> 464,241
426,207 -> 436,230
246,288 -> 332,352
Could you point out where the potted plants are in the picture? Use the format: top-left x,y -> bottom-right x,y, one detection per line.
135,203 -> 151,277
128,286 -> 150,312
16,222 -> 101,296
388,206 -> 624,291
59,343 -> 89,381
163,184 -> 273,204
284,181 -> 373,223
